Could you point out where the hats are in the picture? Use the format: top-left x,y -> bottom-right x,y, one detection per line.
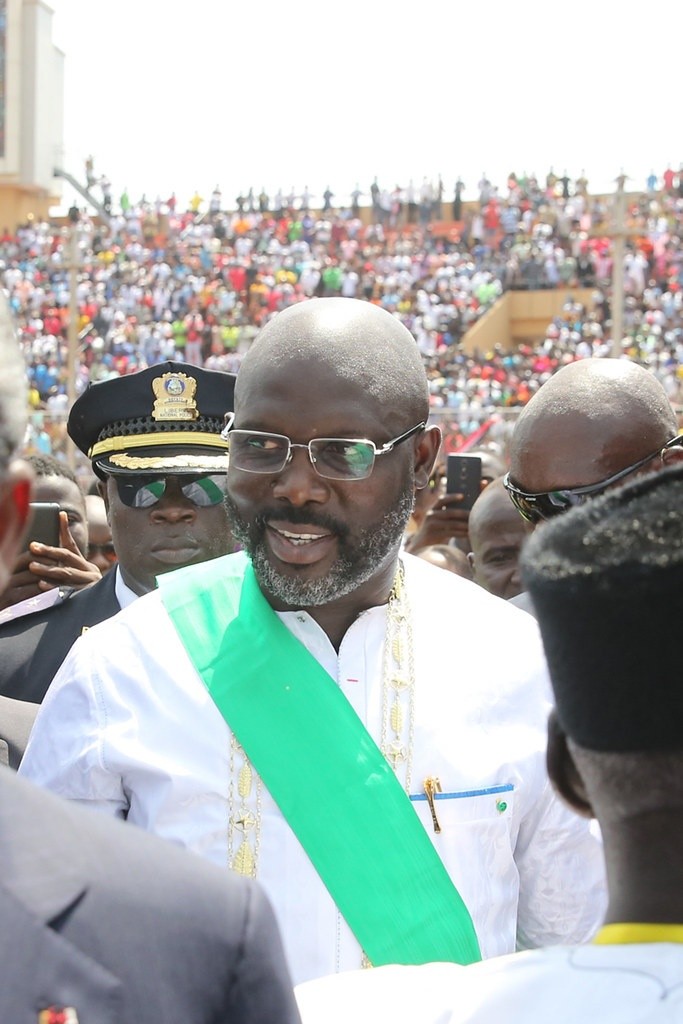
66,359 -> 238,476
520,461 -> 683,753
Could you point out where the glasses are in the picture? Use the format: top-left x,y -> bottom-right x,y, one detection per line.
219,420 -> 424,482
113,473 -> 226,508
86,544 -> 118,561
502,435 -> 683,525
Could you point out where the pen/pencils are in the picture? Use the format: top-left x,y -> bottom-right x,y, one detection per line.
425,776 -> 442,834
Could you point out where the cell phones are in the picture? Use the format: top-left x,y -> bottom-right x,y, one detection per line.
18,502 -> 60,574
446,453 -> 482,512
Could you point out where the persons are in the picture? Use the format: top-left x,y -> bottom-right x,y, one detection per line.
0,299 -> 304,1024
295,464 -> 683,1024
17,297 -> 609,983
0,155 -> 683,792
